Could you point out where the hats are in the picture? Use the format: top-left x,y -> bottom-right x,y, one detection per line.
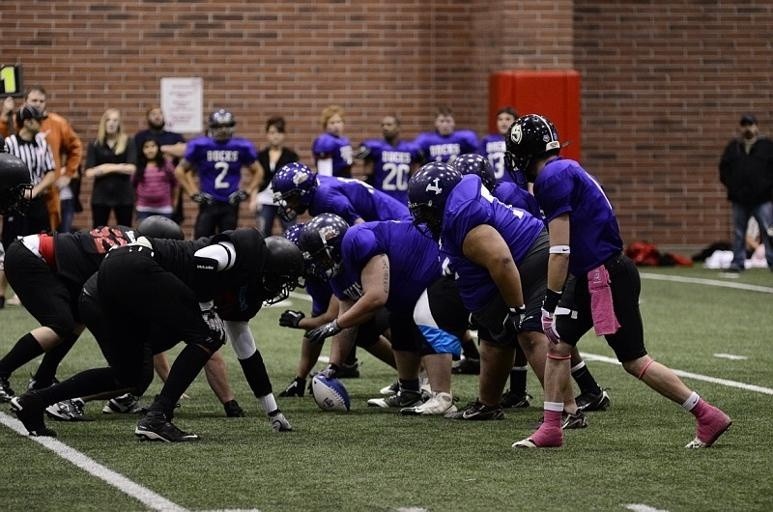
15,104 -> 47,121
740,115 -> 755,124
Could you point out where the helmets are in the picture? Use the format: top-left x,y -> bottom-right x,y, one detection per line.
208,110 -> 235,127
263,235 -> 307,305
270,161 -> 318,223
136,215 -> 183,240
286,212 -> 350,284
406,112 -> 562,241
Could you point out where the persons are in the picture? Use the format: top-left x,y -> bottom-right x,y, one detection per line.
1,88 -> 608,441
503,112 -> 732,450
718,114 -> 773,276
744,214 -> 762,260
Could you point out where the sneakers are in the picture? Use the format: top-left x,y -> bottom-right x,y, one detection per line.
684,405 -> 732,449
341,357 -> 610,430
0,376 -> 197,443
6,294 -> 21,305
511,422 -> 562,449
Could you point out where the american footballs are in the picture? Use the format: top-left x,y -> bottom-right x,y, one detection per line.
313,376 -> 350,412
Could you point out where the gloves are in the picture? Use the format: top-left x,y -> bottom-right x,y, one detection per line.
278,309 -> 341,398
223,399 -> 244,417
266,407 -> 292,433
228,190 -> 248,206
540,305 -> 561,346
198,298 -> 226,344
501,303 -> 526,333
191,192 -> 213,207
